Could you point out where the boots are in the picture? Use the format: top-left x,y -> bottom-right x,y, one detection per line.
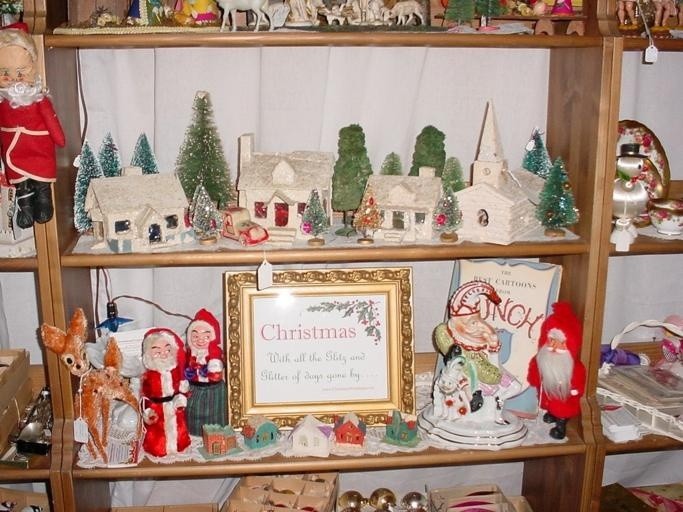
16,181 -> 35,229
34,179 -> 55,225
543,411 -> 555,424
550,417 -> 569,439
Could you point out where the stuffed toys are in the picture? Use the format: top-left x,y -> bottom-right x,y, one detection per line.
610,143 -> 648,252
41,306 -> 150,468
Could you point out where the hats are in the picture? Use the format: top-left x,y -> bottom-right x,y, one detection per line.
140,326 -> 184,371
614,142 -> 651,161
449,280 -> 501,318
0,21 -> 39,60
537,301 -> 582,360
185,306 -> 221,350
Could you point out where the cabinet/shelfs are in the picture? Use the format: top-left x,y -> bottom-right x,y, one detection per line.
33,0 -> 615,512
590,0 -> 683,512
0,0 -> 65,512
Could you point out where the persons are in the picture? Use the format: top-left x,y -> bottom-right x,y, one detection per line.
527,303 -> 587,440
0,21 -> 65,228
139,328 -> 191,456
185,307 -> 229,437
